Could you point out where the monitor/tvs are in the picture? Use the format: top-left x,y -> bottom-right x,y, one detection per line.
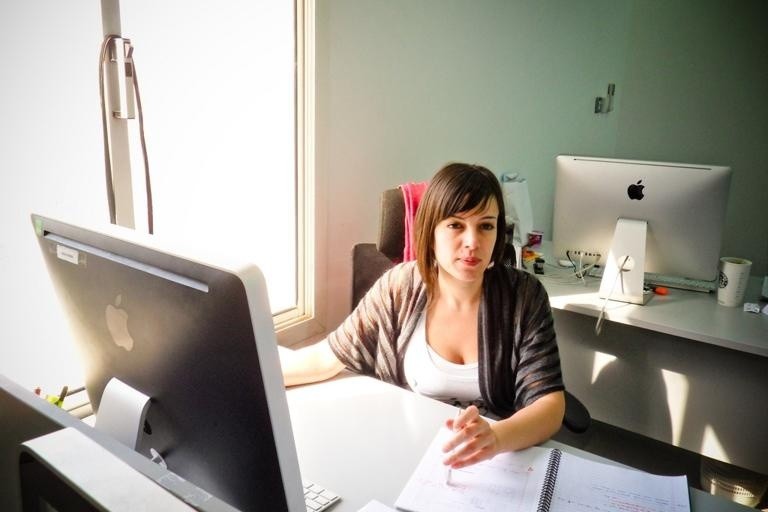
551,154 -> 733,306
29,210 -> 305,512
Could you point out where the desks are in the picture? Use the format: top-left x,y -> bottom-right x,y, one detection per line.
1,336 -> 768,512
480,236 -> 768,363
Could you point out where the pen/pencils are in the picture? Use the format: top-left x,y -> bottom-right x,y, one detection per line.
35,385 -> 68,408
447,406 -> 463,485
644,283 -> 671,294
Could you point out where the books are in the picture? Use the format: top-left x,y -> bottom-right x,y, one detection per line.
393,420 -> 691,512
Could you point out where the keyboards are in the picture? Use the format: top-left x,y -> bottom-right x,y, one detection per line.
584,268 -> 717,293
302,481 -> 342,512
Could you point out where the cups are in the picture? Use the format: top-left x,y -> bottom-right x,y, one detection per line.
717,256 -> 752,308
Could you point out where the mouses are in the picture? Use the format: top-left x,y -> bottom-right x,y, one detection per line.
558,260 -> 575,268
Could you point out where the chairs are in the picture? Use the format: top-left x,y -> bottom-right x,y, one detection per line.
351,181 -> 403,314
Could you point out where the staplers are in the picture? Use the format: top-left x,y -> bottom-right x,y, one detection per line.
533,258 -> 545,274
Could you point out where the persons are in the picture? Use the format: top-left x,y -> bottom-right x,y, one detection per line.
281,161 -> 567,469
